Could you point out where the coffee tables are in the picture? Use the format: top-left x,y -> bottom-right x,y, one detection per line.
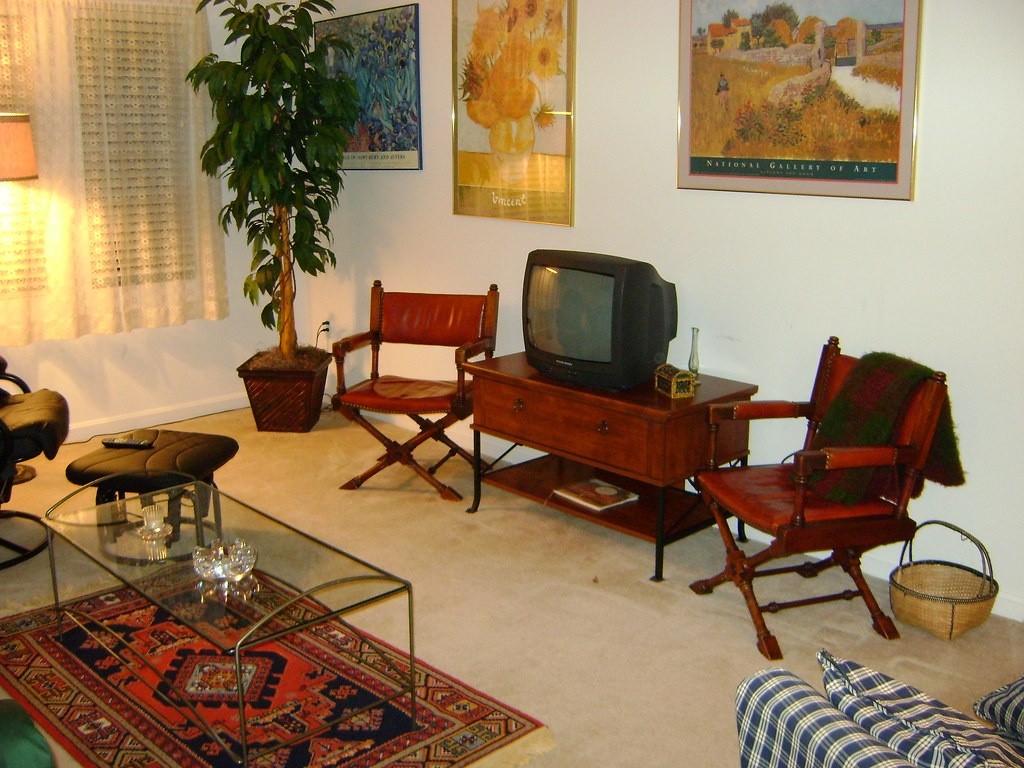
39,469 -> 416,768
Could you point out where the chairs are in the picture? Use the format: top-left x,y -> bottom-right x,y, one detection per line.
689,337 -> 966,661
331,280 -> 501,502
0,354 -> 70,570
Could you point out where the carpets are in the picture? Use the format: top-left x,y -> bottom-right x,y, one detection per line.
0,546 -> 559,768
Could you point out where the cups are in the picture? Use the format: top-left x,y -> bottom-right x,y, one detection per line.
142,504 -> 165,533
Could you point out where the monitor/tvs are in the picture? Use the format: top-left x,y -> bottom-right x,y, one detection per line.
522,249 -> 678,393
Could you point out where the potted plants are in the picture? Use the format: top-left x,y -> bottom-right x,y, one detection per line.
183,0 -> 364,434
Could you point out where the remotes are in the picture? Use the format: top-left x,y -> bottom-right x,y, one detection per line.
102,438 -> 152,450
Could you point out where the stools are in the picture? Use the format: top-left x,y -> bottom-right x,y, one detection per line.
65,427 -> 240,566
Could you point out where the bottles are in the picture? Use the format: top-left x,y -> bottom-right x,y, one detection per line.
689,327 -> 700,378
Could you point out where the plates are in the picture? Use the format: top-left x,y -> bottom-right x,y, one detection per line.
137,524 -> 173,541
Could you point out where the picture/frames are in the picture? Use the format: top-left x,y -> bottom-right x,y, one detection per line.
676,0 -> 923,201
451,0 -> 577,227
313,2 -> 423,171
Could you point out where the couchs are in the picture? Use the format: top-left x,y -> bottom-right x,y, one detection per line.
735,647 -> 1024,768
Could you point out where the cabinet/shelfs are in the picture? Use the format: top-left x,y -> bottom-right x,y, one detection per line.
462,350 -> 760,582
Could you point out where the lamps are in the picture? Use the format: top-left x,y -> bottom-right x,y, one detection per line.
0,112 -> 40,485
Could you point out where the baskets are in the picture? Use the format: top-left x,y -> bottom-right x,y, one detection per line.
888,519 -> 1000,643
